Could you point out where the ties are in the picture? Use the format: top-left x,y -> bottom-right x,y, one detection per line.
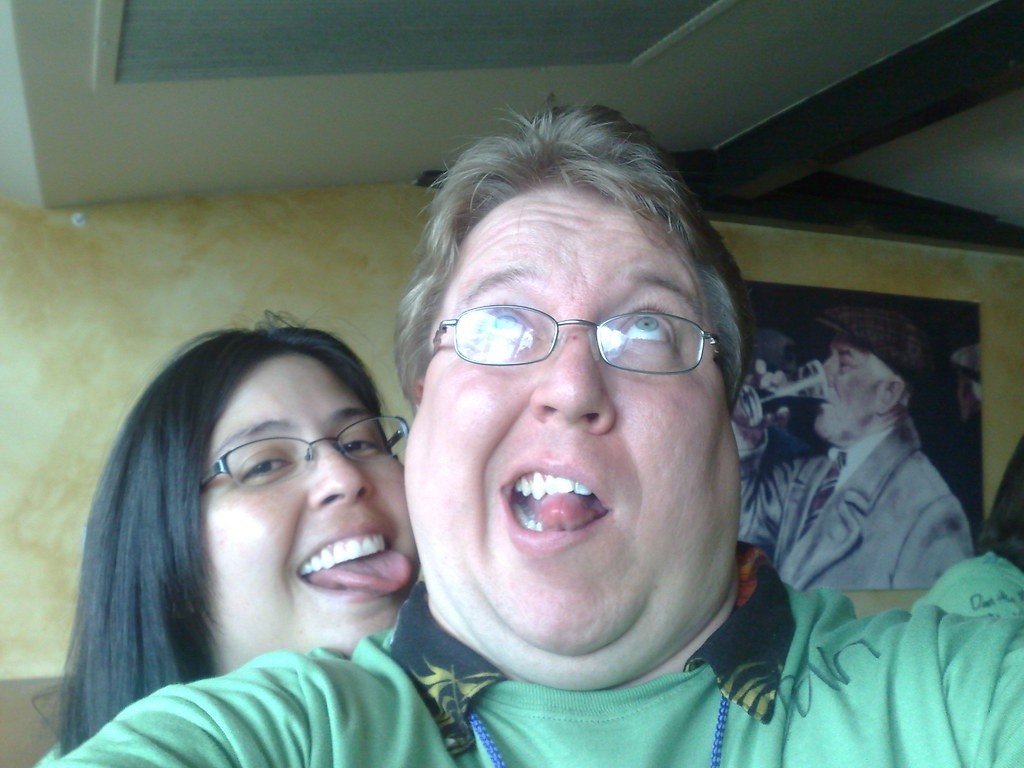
798,451 -> 847,539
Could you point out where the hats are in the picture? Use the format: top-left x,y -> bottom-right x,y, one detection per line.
820,304 -> 928,380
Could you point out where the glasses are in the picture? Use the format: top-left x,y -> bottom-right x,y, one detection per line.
196,414 -> 410,493
424,303 -> 723,376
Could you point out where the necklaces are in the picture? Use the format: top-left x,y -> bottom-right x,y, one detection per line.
461,683 -> 731,768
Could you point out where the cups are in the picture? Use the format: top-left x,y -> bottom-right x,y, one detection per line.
737,360 -> 830,428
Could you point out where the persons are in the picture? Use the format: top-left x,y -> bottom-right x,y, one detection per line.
911,437 -> 1024,621
50,108 -> 1022,768
29,325 -> 423,768
